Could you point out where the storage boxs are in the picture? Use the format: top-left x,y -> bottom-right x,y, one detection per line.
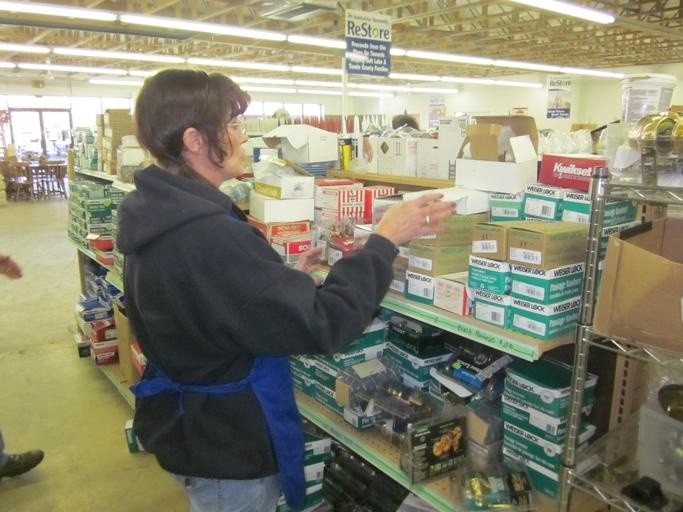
219,108 -> 682,511
68,109 -> 155,454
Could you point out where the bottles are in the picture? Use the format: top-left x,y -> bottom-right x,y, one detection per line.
587,165 -> 609,199
335,133 -> 373,169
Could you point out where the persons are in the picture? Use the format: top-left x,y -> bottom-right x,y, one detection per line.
392,114 -> 419,131
0,252 -> 45,480
115,69 -> 457,512
272,108 -> 290,126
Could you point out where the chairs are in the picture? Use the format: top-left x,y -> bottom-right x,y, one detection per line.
0,152 -> 67,203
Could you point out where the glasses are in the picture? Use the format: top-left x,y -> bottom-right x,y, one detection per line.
226,114 -> 249,134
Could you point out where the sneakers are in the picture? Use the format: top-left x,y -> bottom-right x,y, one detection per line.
0,448 -> 46,480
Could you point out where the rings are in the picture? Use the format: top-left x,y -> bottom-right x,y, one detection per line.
426,215 -> 430,224
